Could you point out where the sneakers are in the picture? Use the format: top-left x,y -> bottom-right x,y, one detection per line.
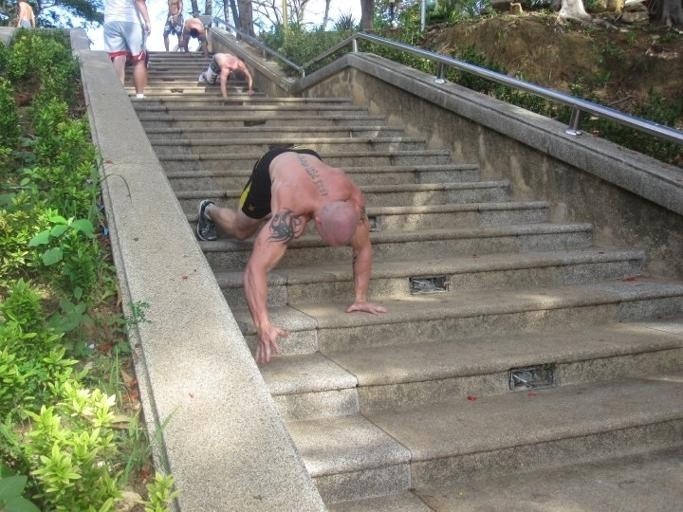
199,72 -> 206,83
196,198 -> 217,242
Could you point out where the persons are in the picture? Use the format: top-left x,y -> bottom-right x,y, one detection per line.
196,146 -> 387,364
102,0 -> 152,100
16,0 -> 36,28
198,52 -> 256,99
178,17 -> 208,53
162,1 -> 184,54
